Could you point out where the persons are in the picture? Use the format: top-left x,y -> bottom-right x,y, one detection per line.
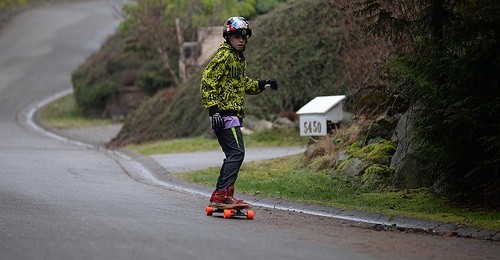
199,17 -> 278,206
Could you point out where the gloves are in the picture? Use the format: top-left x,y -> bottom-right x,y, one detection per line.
259,79 -> 278,91
212,113 -> 225,131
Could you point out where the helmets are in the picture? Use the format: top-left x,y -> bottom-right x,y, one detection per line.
223,16 -> 252,39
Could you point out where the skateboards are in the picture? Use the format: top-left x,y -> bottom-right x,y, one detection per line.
204,203 -> 255,219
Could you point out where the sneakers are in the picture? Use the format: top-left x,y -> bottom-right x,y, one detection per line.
209,187 -> 248,206
